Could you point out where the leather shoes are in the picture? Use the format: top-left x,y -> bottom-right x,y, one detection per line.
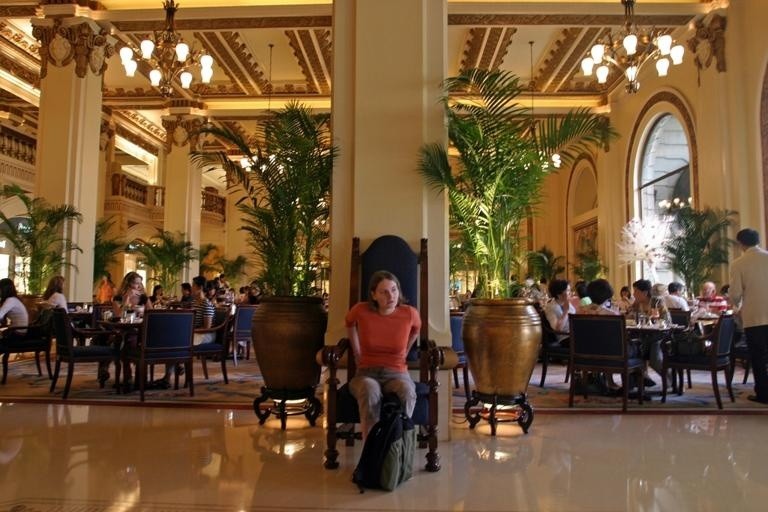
746,395 -> 767,405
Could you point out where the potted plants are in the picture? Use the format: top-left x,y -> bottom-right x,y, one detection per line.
0,182 -> 83,326
185,99 -> 343,399
125,226 -> 199,309
92,214 -> 136,321
412,68 -> 622,395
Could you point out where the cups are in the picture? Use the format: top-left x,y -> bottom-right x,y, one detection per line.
637,312 -> 647,329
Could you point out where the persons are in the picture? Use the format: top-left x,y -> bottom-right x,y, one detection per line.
0,268 -> 267,389
724,228 -> 768,406
345,268 -> 422,445
538,273 -> 730,404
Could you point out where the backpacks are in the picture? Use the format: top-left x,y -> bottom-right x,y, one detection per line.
351,412 -> 415,491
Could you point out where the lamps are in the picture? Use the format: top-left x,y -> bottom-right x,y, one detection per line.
580,1 -> 685,95
118,0 -> 213,97
510,41 -> 562,172
240,43 -> 292,176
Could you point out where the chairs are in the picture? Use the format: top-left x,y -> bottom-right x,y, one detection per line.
316,237 -> 459,473
